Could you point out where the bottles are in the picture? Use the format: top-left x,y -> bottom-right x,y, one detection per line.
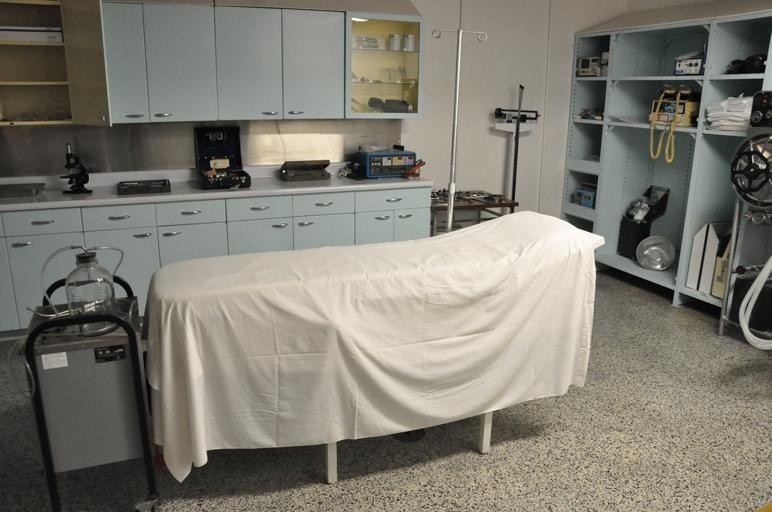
389,34 -> 400,50
404,34 -> 416,53
65,252 -> 118,336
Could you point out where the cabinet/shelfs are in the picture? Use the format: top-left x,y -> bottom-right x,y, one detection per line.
215,5 -> 345,121
99,0 -> 219,127
226,192 -> 355,255
347,11 -> 425,119
0,0 -> 71,126
0,208 -> 88,331
355,188 -> 431,245
561,1 -> 772,336
81,199 -> 229,318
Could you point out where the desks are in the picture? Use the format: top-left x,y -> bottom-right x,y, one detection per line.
432,190 -> 519,238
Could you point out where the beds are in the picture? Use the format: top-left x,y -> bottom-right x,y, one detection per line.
141,210 -> 605,484
